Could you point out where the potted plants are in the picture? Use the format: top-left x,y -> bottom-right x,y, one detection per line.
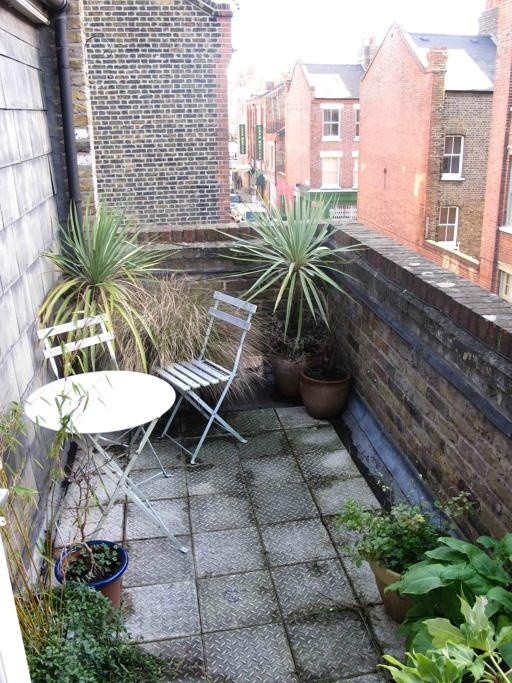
51,384 -> 128,611
334,472 -> 476,623
216,185 -> 352,419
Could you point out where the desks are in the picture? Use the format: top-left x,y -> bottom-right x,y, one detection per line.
23,367 -> 190,555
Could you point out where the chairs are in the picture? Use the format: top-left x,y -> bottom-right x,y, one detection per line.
37,316 -> 170,492
153,293 -> 257,464
